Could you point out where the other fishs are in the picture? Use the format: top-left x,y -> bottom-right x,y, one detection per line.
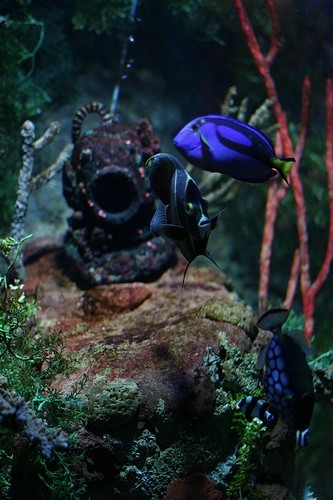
237,308 -> 314,449
145,153 -> 228,291
173,115 -> 297,186
